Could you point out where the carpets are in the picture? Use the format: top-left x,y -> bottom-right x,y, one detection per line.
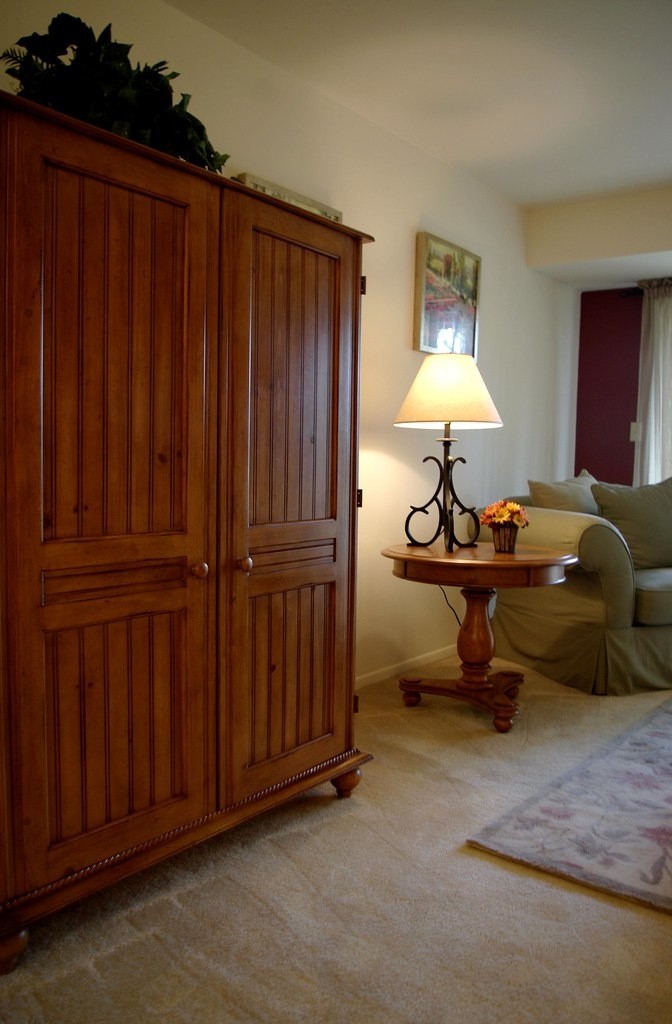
464,692 -> 672,917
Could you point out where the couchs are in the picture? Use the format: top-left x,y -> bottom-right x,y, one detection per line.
469,469 -> 672,697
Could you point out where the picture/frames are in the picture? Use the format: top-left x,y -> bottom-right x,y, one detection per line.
412,231 -> 482,363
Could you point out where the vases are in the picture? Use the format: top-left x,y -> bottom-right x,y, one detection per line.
490,520 -> 519,552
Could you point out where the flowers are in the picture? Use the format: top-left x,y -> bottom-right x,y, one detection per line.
478,500 -> 531,529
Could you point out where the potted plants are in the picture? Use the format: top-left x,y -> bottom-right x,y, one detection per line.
0,13 -> 229,181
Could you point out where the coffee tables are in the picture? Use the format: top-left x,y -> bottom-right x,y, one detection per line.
380,540 -> 580,735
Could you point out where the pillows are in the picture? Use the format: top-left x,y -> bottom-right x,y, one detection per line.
527,468 -> 605,517
590,477 -> 672,571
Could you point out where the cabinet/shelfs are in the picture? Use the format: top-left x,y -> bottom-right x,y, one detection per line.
0,89 -> 375,977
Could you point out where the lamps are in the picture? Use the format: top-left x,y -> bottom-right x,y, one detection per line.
393,353 -> 504,554
